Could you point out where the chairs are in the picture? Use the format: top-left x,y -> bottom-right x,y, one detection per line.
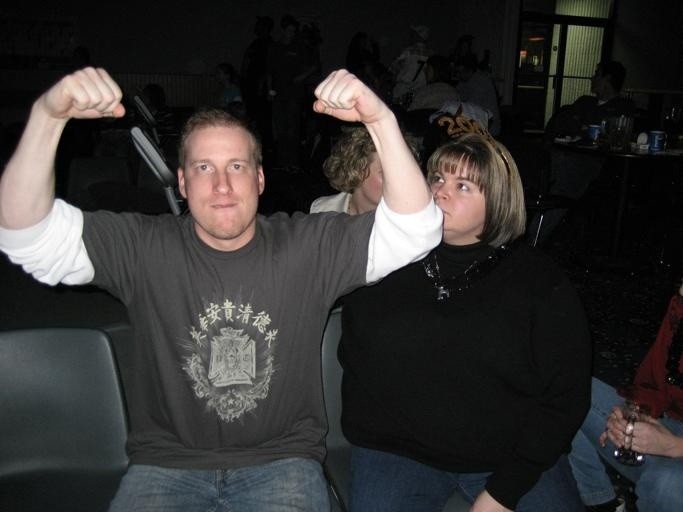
322,303 -> 475,511
0,326 -> 130,511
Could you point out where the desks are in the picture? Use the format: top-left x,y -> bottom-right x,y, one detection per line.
536,137 -> 681,287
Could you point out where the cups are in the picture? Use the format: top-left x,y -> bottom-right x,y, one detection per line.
612,400 -> 652,465
588,125 -> 599,145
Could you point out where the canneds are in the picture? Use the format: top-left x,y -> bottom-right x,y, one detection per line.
588,124 -> 601,142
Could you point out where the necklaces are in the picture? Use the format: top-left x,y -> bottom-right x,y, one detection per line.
421,242 -> 501,303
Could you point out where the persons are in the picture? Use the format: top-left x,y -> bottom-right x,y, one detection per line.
544,57 -> 639,137
0,65 -> 442,511
565,284 -> 682,510
333,133 -> 592,511
306,121 -> 424,215
69,15 -> 503,131
